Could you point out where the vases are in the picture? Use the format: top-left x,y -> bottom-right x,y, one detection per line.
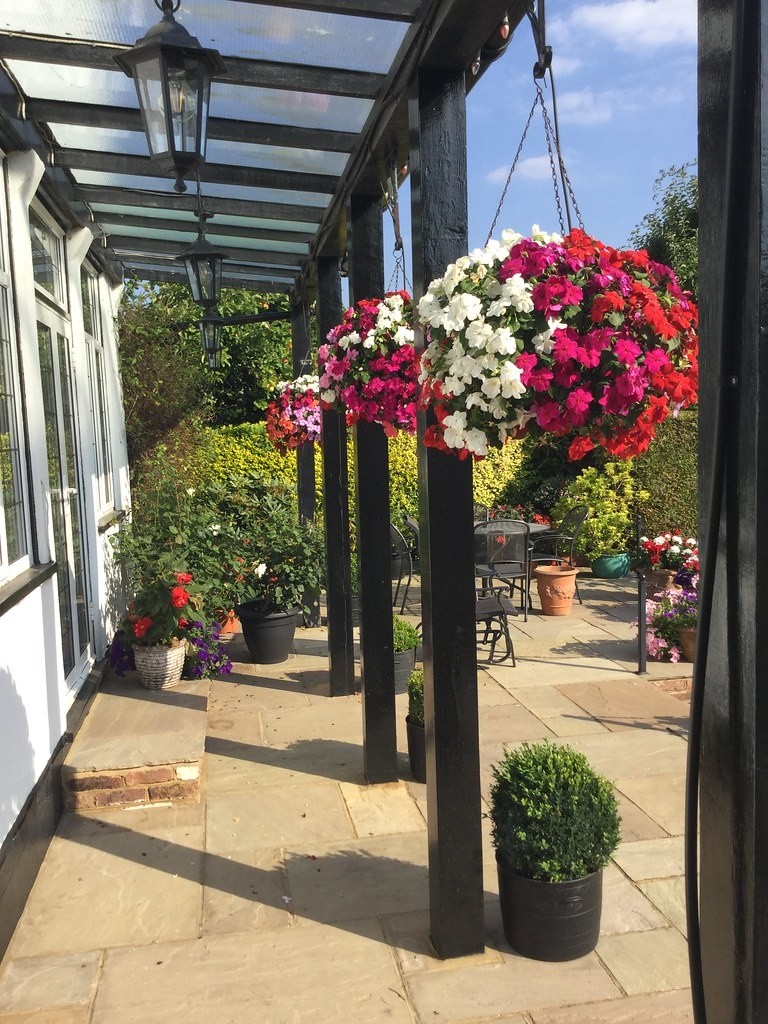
644,568 -> 681,601
131,634 -> 187,689
533,566 -> 579,616
215,608 -> 238,635
676,625 -> 697,662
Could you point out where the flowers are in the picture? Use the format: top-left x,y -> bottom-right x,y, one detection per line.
318,289 -> 419,438
111,458 -> 260,681
638,533 -> 701,572
645,564 -> 700,665
414,224 -> 698,461
264,375 -> 324,457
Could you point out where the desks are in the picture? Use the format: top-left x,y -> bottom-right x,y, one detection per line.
470,520 -> 548,597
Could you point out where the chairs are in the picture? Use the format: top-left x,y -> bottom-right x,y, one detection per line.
390,512 -> 420,615
473,518 -> 535,622
524,505 -> 590,603
475,586 -> 519,666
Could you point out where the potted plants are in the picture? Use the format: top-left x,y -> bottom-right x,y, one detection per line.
349,552 -> 360,628
403,668 -> 428,784
229,507 -> 327,663
481,735 -> 623,962
392,606 -> 420,694
551,460 -> 651,579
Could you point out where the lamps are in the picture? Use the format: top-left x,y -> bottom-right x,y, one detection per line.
112,0 -> 228,191
199,292 -> 227,374
174,162 -> 230,313
